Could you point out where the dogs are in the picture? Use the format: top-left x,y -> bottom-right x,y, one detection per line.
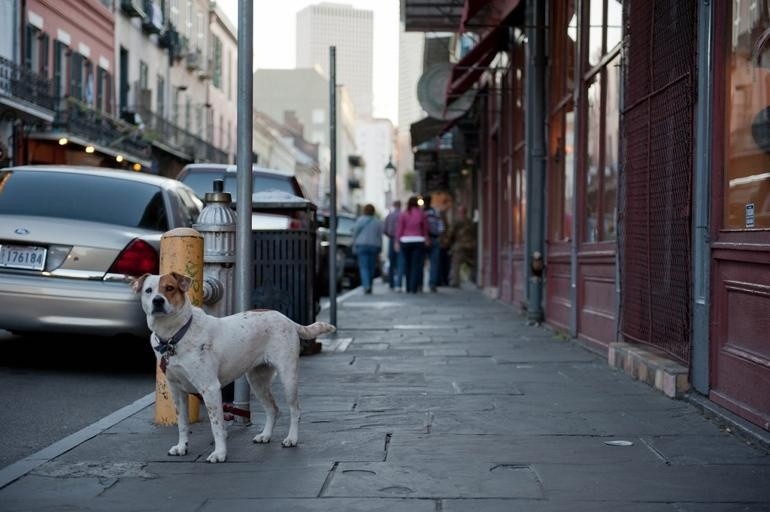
128,271 -> 337,463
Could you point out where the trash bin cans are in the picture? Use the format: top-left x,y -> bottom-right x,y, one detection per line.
231,191 -> 321,356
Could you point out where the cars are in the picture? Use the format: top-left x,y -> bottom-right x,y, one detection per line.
171,158 -> 361,295
0,159 -> 211,350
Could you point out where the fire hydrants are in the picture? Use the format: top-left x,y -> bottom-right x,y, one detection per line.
187,169 -> 237,317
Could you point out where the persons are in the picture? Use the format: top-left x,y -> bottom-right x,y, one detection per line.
351,195 -> 443,293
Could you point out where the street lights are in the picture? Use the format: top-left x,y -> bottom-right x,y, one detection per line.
382,152 -> 399,216
